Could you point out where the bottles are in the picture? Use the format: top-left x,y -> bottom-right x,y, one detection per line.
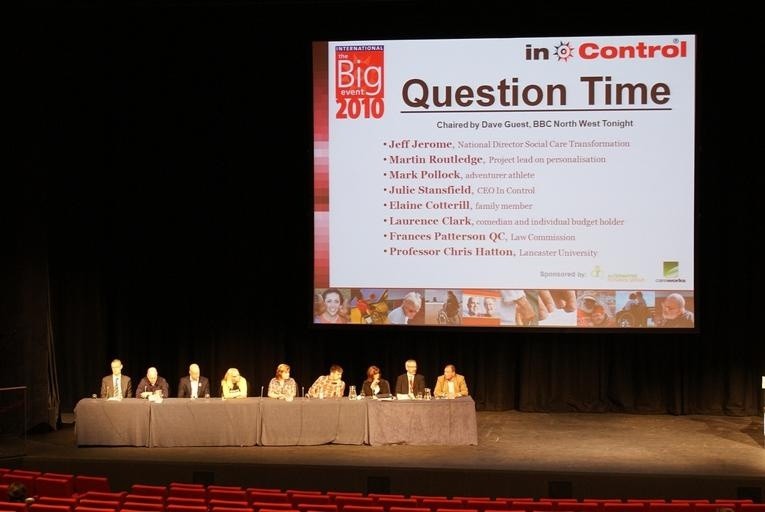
424,388 -> 432,400
348,386 -> 357,400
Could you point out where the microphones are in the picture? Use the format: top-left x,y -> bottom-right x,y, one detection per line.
106,386 -> 109,398
144,386 -> 147,398
261,386 -> 264,397
302,385 -> 305,398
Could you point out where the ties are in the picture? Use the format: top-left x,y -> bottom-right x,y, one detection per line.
409,376 -> 414,394
115,377 -> 119,396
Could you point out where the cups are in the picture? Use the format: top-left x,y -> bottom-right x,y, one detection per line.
417,392 -> 423,400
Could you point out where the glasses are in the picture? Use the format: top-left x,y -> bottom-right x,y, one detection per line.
661,302 -> 680,310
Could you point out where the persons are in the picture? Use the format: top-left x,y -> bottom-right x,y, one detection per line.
361,365 -> 391,396
100,359 -> 133,398
177,363 -> 211,398
314,287 -> 695,328
395,359 -> 425,397
267,364 -> 296,402
433,363 -> 468,398
220,368 -> 248,400
308,364 -> 346,398
135,366 -> 169,399
